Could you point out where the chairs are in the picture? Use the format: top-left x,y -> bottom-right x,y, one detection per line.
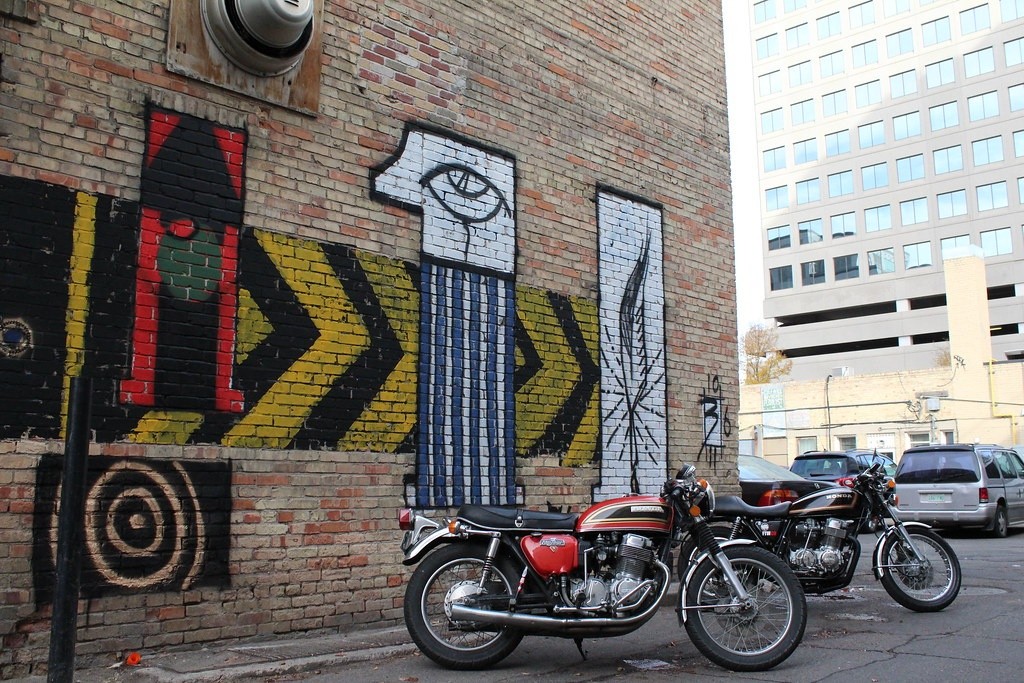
943,462 -> 962,469
911,459 -> 931,470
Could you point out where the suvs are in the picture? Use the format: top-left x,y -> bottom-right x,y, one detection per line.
789,449 -> 897,530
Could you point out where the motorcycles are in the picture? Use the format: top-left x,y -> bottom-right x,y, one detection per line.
676,450 -> 963,612
396,460 -> 808,671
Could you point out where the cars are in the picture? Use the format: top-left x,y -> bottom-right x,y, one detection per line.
735,453 -> 842,538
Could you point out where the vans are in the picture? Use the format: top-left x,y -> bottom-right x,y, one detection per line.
894,443 -> 1023,537
